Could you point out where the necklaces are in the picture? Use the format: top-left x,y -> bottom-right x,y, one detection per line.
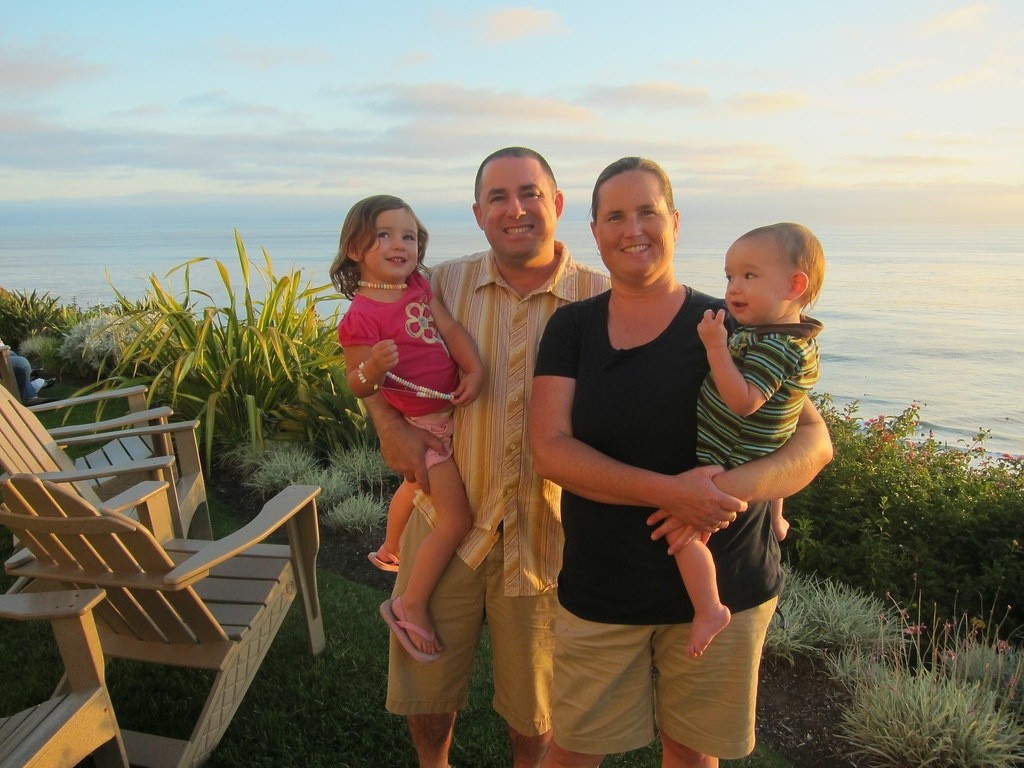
358,361 -> 454,400
357,281 -> 407,290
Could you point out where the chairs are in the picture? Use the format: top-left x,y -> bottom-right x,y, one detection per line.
0,340 -> 325,768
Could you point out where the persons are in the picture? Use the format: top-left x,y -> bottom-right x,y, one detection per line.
365,148 -> 612,768
528,157 -> 835,768
667,223 -> 826,658
329,194 -> 486,662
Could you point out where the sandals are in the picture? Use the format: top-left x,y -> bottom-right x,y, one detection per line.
367,544 -> 400,572
379,595 -> 443,663
